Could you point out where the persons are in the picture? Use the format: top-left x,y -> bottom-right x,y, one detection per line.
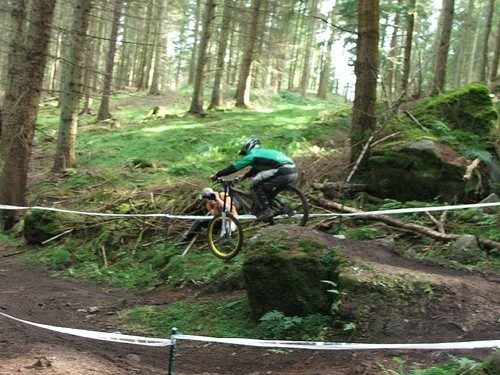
175,188 -> 237,246
211,138 -> 298,221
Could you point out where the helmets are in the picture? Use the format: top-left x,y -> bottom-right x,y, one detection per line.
242,139 -> 261,152
201,188 -> 215,197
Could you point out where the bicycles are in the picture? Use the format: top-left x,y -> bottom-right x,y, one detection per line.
207,175 -> 309,259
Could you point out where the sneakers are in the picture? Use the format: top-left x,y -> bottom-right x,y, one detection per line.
254,208 -> 277,226
